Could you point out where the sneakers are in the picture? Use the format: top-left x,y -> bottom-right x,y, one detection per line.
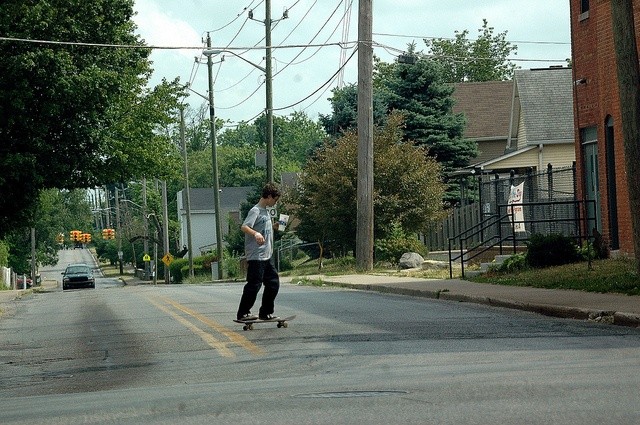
237,314 -> 258,321
259,314 -> 280,321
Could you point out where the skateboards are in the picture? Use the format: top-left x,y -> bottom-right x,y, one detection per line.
233,314 -> 297,330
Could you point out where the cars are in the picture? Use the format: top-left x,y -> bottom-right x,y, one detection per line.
17,275 -> 33,288
61,264 -> 95,290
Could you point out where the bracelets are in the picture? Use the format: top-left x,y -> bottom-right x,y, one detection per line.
255,233 -> 261,237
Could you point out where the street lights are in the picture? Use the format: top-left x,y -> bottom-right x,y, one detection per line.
102,206 -> 138,272
121,198 -> 153,283
92,207 -> 112,229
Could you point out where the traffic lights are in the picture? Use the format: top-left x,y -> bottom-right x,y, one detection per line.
108,228 -> 112,238
73,231 -> 78,240
103,228 -> 107,238
70,230 -> 73,240
112,229 -> 115,238
79,231 -> 82,239
88,234 -> 91,241
85,234 -> 88,241
82,234 -> 85,241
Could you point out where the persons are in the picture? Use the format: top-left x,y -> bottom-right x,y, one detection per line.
236,183 -> 287,322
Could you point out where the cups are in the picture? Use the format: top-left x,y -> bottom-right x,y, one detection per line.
278,214 -> 289,232
